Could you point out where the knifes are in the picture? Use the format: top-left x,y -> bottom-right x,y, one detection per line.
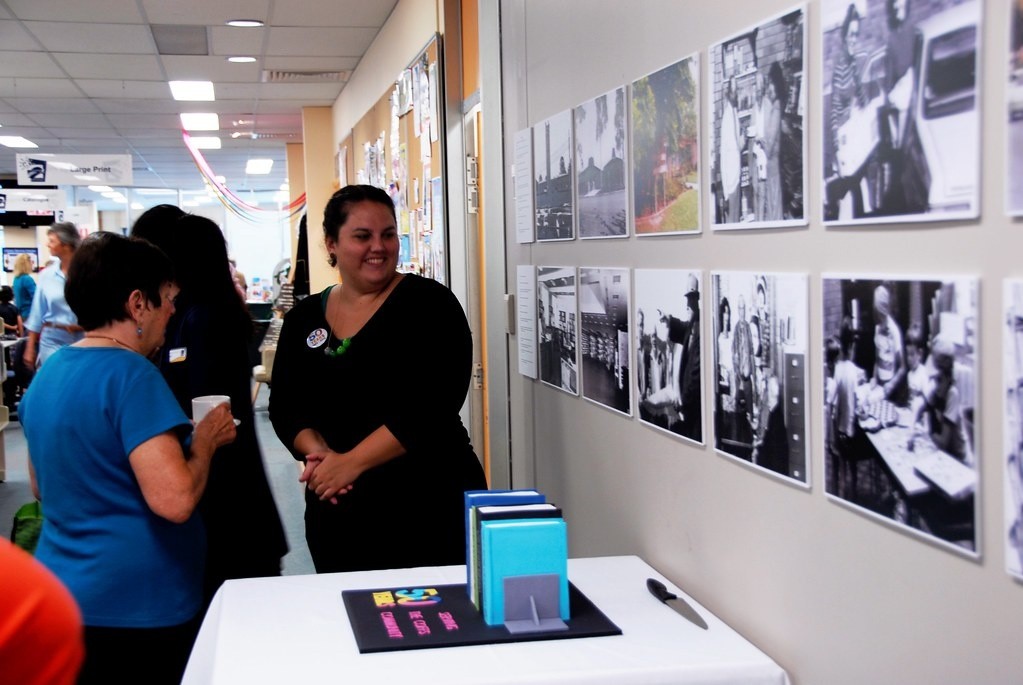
647,578 -> 708,631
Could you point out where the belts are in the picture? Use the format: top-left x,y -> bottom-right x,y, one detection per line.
44,322 -> 83,334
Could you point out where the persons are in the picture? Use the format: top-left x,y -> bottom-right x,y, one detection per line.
0,253 -> 37,421
717,57 -> 808,226
126,204 -> 290,579
225,258 -> 249,301
16,231 -> 237,685
538,301 -> 550,349
827,0 -> 927,220
831,282 -> 969,524
720,279 -> 793,458
23,221 -> 86,372
635,273 -> 699,423
268,185 -> 514,579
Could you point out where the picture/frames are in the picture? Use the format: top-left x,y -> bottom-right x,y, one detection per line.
824,272 -> 986,562
1004,0 -> 1023,218
578,266 -> 634,418
536,265 -> 580,397
629,51 -> 704,238
708,2 -> 809,231
574,84 -> 629,240
634,268 -> 707,446
533,106 -> 575,244
818,0 -> 987,228
999,279 -> 1023,582
713,270 -> 809,491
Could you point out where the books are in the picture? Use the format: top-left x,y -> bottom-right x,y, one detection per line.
463,488 -> 576,627
278,280 -> 294,312
263,315 -> 284,347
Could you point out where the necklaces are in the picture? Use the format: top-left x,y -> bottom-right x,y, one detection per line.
323,296 -> 365,356
83,335 -> 139,352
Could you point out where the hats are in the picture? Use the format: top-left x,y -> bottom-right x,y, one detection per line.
684,274 -> 699,295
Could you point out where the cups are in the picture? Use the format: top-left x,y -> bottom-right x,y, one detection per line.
191,394 -> 230,425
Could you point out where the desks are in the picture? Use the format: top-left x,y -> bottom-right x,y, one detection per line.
180,556 -> 792,685
